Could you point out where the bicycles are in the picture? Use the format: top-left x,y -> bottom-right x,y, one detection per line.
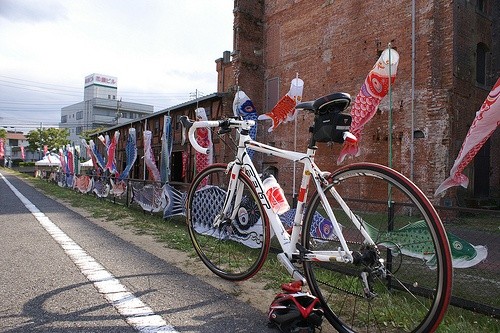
184,92 -> 455,333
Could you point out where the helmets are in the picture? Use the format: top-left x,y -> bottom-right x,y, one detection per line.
266,291 -> 322,329
